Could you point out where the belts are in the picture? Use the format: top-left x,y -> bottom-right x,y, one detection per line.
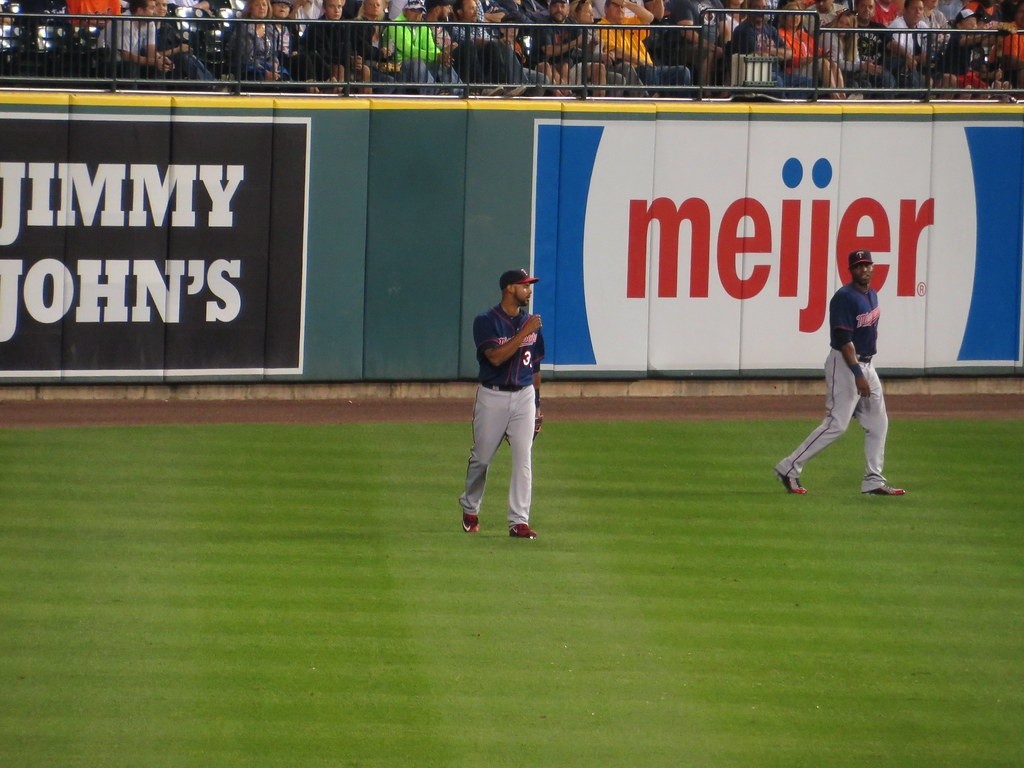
482,383 -> 522,392
858,355 -> 872,363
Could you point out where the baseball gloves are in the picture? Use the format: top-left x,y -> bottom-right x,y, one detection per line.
503,414 -> 544,447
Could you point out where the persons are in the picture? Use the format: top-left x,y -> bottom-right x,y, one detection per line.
774,250 -> 906,495
0,0 -> 1024,104
459,268 -> 545,540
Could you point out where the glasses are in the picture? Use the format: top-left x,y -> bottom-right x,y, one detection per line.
857,262 -> 872,268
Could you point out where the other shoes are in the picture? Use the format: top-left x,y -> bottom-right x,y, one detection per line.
482,86 -> 503,95
505,85 -> 528,97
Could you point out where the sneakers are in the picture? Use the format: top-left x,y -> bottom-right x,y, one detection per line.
462,511 -> 479,532
509,524 -> 537,538
776,467 -> 807,494
863,484 -> 905,495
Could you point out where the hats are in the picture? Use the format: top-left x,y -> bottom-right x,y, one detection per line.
270,0 -> 293,14
848,249 -> 872,269
955,9 -> 982,24
500,268 -> 539,289
404,0 -> 426,11
549,0 -> 569,4
